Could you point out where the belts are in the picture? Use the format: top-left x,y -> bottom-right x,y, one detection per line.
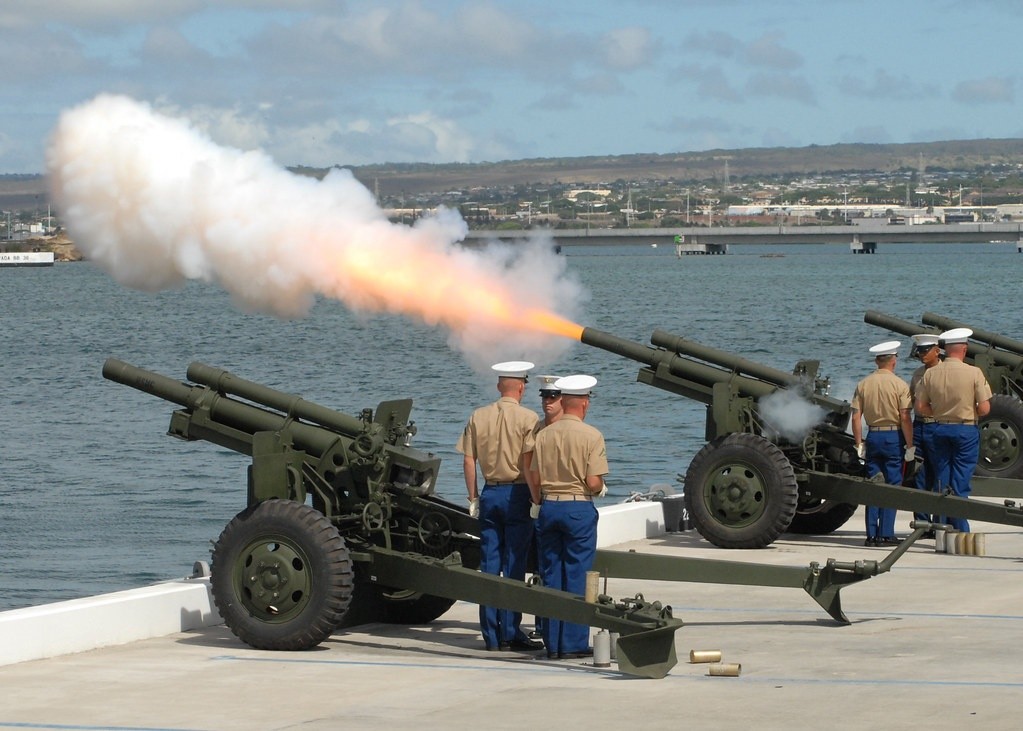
542,494 -> 593,502
937,419 -> 979,425
486,477 -> 526,484
869,426 -> 901,431
914,416 -> 935,423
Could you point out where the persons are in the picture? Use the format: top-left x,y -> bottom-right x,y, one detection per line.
454,360 -> 609,659
850,327 -> 993,547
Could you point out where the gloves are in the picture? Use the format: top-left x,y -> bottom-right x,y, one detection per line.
852,442 -> 866,460
592,483 -> 608,499
904,445 -> 916,462
529,499 -> 541,519
467,496 -> 479,520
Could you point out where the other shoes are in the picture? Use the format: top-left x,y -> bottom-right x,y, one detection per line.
486,644 -> 499,651
548,643 -> 595,658
866,535 -> 904,546
501,633 -> 543,650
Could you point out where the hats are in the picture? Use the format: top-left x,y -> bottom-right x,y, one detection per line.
939,328 -> 974,345
556,374 -> 598,399
868,341 -> 902,357
536,375 -> 565,398
492,361 -> 534,384
912,334 -> 939,352
